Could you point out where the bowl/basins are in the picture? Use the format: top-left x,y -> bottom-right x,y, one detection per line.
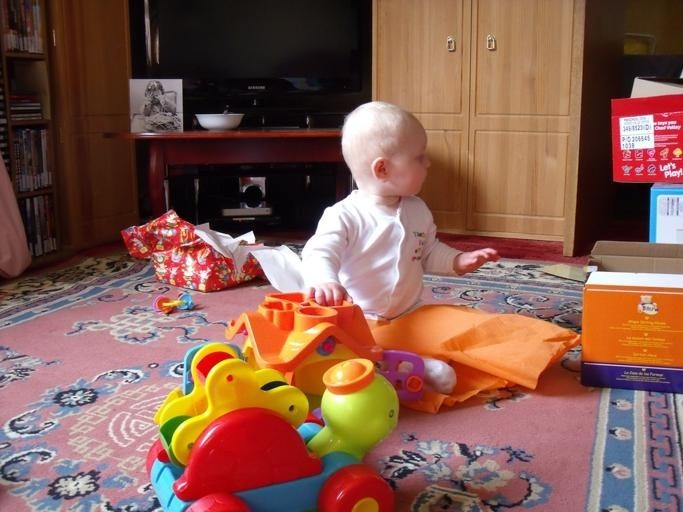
194,113 -> 245,131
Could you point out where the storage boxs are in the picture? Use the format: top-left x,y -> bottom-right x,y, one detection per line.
580,239 -> 683,394
610,75 -> 683,183
649,183 -> 683,243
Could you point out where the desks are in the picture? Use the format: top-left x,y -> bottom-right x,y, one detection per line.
104,131 -> 350,219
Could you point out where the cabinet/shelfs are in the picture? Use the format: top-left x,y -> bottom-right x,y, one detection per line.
0,0 -> 69,264
372,1 -> 586,256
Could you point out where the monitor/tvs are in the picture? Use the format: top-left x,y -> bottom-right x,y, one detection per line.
142,0 -> 372,117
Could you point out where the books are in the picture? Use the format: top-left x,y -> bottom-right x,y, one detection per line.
0,0 -> 57,258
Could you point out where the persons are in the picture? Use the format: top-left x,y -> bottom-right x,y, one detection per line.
301,100 -> 501,394
140,80 -> 177,117
